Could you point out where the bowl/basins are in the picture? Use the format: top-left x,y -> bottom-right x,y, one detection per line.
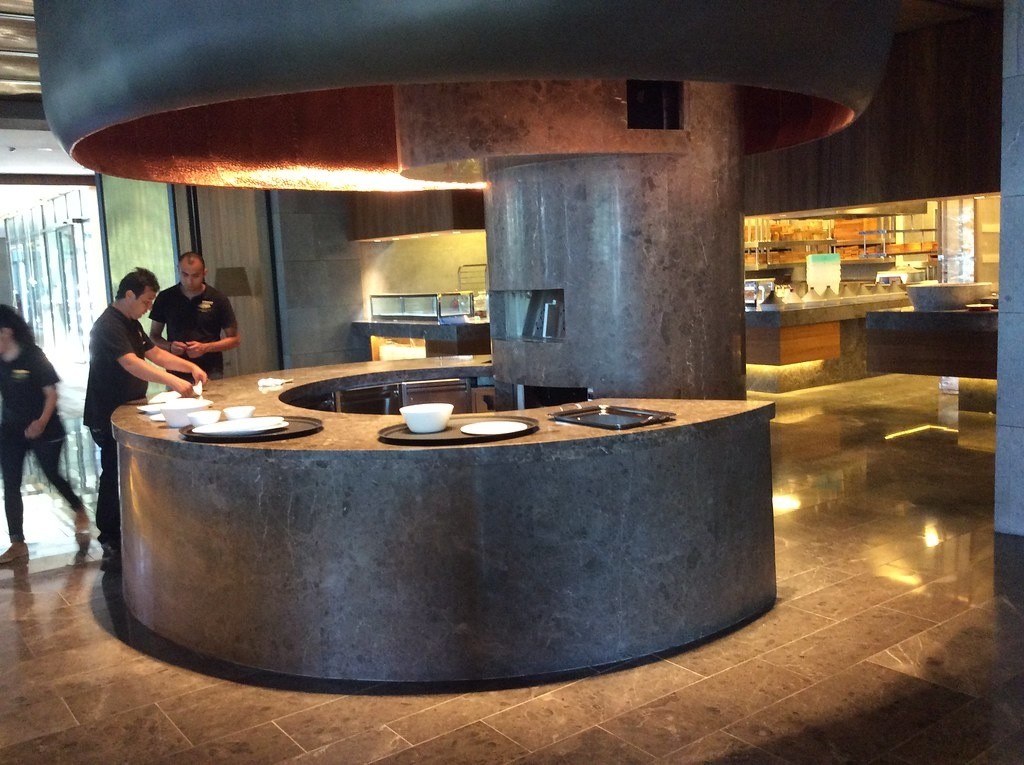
223,405 -> 255,420
160,398 -> 215,428
187,409 -> 221,427
399,402 -> 454,434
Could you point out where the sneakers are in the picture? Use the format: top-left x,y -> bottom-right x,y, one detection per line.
74,503 -> 89,529
0,543 -> 29,563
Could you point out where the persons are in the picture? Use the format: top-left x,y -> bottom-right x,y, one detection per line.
148,251 -> 241,390
0,304 -> 87,564
83,268 -> 208,551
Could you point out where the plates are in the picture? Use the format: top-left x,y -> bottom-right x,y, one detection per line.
150,414 -> 166,421
460,421 -> 528,436
136,404 -> 161,414
191,416 -> 290,437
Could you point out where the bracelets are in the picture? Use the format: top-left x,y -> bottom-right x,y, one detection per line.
169,343 -> 172,352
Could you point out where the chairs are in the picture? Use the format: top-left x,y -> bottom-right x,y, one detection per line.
401,378 -> 473,414
335,383 -> 400,416
